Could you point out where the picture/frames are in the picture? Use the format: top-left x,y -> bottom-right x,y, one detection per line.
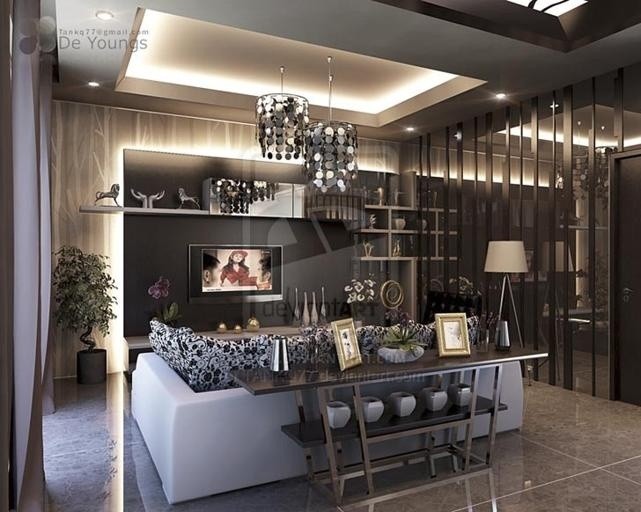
331,316 -> 365,371
435,312 -> 471,357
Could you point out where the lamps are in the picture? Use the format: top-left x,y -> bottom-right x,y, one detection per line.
252,65 -> 309,161
540,243 -> 574,345
554,154 -> 587,198
483,240 -> 528,347
596,145 -> 616,206
307,55 -> 358,193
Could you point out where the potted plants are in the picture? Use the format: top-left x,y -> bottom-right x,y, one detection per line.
49,245 -> 114,382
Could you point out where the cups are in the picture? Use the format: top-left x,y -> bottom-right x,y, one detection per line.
476,328 -> 491,355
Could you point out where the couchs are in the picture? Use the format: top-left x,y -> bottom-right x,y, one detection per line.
126,322 -> 524,504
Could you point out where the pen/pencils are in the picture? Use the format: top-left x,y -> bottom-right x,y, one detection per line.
471,309 -> 499,329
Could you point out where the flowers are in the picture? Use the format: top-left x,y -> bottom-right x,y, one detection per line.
150,276 -> 178,322
380,305 -> 423,358
343,273 -> 376,307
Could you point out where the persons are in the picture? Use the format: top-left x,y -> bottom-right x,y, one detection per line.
203,254 -> 221,287
221,251 -> 250,286
256,254 -> 272,291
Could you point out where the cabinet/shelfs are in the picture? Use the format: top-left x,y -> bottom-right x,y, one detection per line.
229,337 -> 552,512
358,201 -> 413,262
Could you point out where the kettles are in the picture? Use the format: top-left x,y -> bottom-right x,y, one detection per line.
267,336 -> 291,378
494,319 -> 512,352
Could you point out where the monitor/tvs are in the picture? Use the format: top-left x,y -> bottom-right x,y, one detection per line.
187,243 -> 284,305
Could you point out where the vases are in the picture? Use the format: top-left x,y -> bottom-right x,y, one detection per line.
377,344 -> 425,364
